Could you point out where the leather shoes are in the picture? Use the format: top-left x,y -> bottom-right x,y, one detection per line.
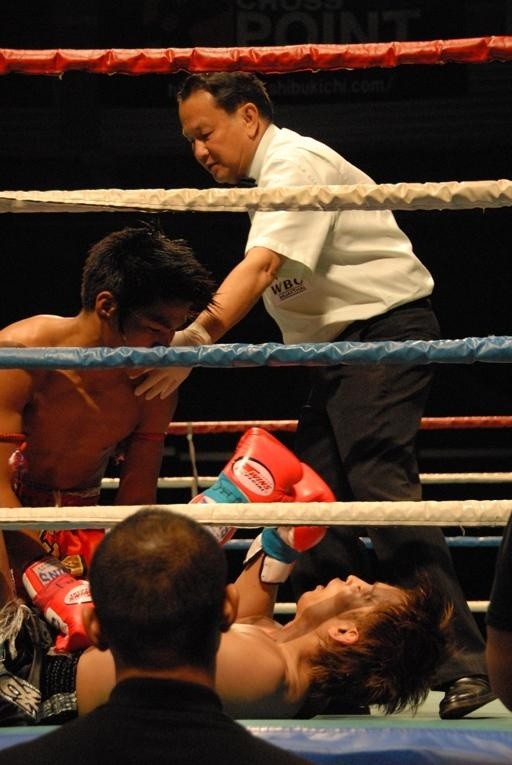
438,673 -> 494,718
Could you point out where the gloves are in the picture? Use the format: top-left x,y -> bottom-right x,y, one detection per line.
260,460 -> 337,561
21,523 -> 107,659
128,323 -> 212,401
185,425 -> 304,550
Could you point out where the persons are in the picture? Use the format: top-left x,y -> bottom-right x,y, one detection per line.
124,70 -> 499,719
481,511 -> 511,716
1,428 -> 456,724
1,220 -> 224,666
1,507 -> 312,765
